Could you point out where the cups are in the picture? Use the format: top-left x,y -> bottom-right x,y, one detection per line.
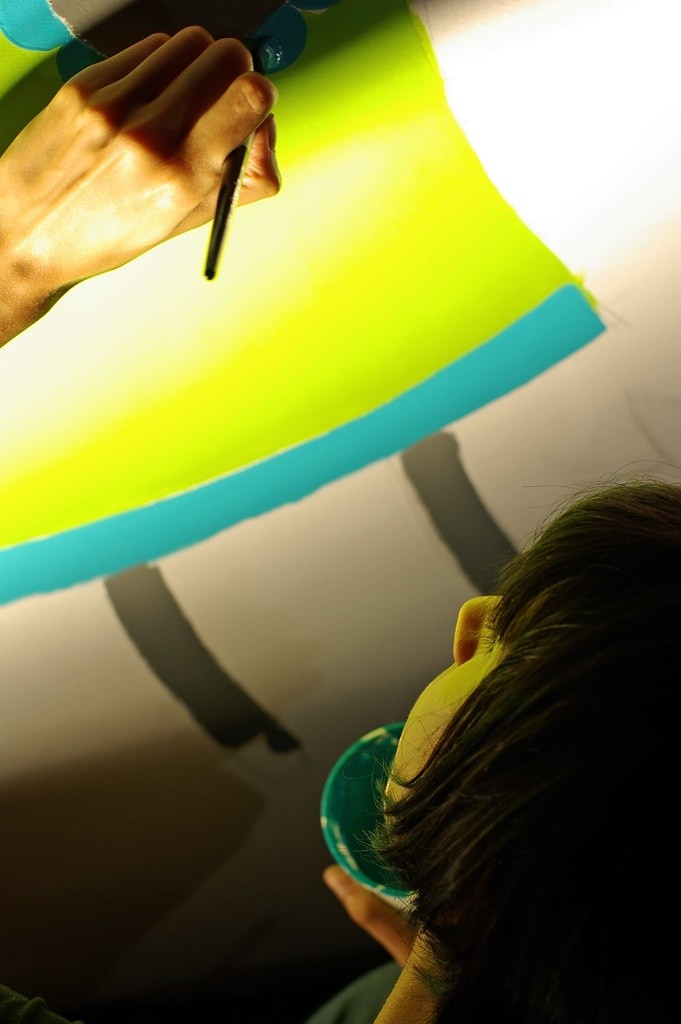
318,726 -> 442,910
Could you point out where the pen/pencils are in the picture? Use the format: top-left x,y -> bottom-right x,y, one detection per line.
204,129 -> 257,279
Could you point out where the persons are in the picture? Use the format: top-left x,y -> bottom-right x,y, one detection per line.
0,26 -> 681,1024
